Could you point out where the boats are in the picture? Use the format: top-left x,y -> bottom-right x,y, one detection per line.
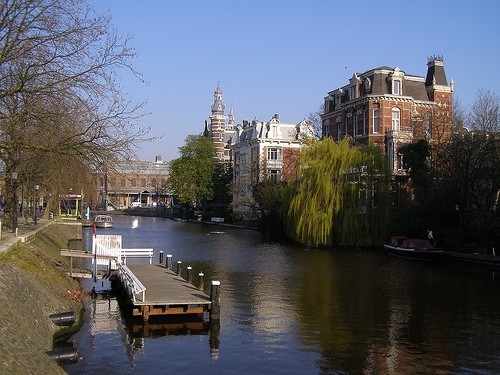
94,215 -> 114,228
383,235 -> 444,260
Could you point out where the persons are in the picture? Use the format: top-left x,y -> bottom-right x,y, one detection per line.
427,228 -> 435,246
